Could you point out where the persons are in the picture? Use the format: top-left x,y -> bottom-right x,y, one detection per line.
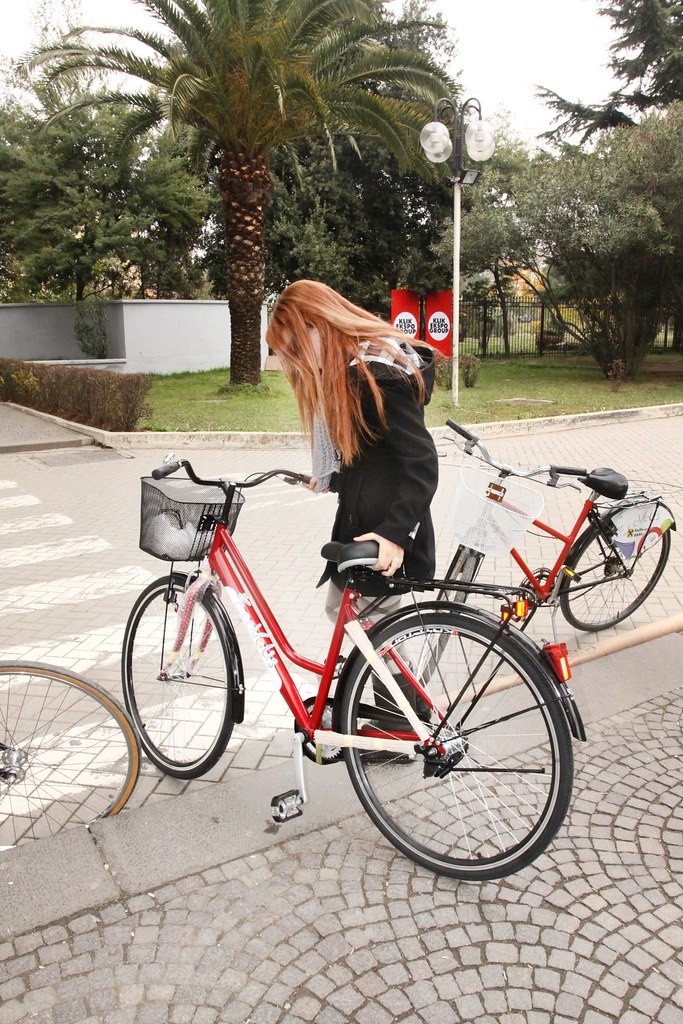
267,280 -> 441,757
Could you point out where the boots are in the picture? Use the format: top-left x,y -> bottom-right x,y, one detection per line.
357,662 -> 417,760
415,677 -> 432,754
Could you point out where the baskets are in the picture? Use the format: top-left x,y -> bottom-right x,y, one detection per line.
448,465 -> 544,558
139,477 -> 246,561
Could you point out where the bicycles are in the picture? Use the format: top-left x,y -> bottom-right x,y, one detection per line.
120,451 -> 587,881
415,418 -> 677,688
0,660 -> 142,853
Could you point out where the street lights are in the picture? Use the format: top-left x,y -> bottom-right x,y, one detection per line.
419,97 -> 496,406
